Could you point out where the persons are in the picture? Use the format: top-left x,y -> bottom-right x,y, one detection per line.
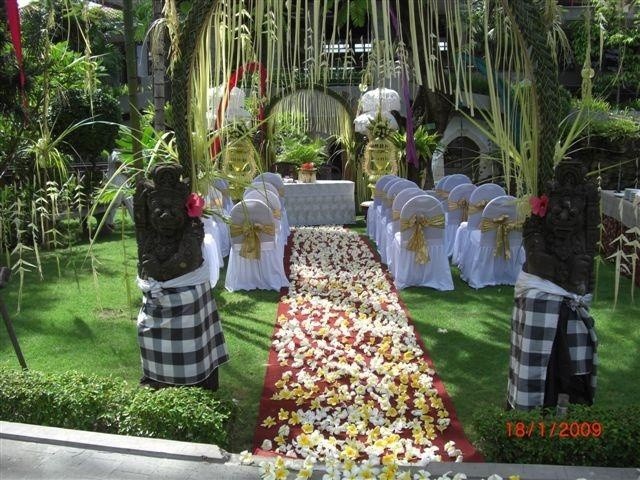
522,158 -> 601,296
133,162 -> 205,281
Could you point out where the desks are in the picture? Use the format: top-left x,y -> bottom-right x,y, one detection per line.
284,179 -> 355,226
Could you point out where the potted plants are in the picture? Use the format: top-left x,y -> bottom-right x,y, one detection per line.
275,134 -> 329,182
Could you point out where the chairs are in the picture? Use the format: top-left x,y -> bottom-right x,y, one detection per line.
367,172 -> 526,292
199,171 -> 290,291
